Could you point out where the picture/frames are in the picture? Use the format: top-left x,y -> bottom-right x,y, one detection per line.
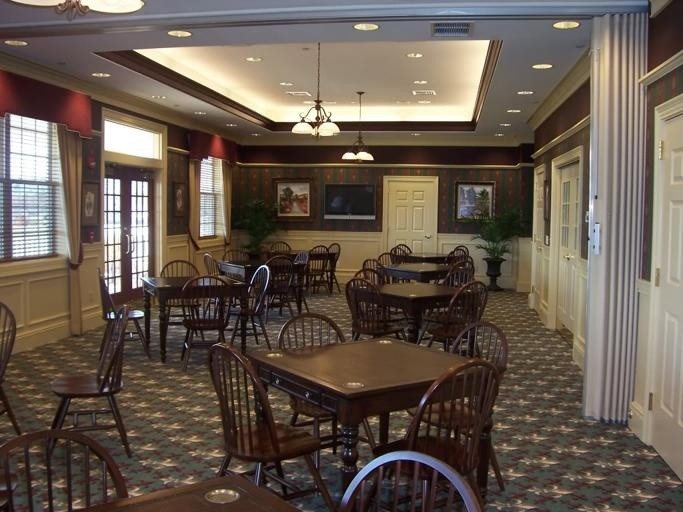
173,182 -> 188,218
272,177 -> 314,223
454,180 -> 496,224
80,141 -> 101,230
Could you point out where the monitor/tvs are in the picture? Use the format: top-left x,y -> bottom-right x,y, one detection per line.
324,183 -> 376,221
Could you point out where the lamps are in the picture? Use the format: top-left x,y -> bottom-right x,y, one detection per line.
291,43 -> 374,161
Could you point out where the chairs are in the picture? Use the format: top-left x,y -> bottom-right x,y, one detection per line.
97,240 -> 342,373
345,244 -> 489,351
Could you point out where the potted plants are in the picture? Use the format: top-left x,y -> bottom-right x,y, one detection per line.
231,197 -> 276,259
458,188 -> 530,292
